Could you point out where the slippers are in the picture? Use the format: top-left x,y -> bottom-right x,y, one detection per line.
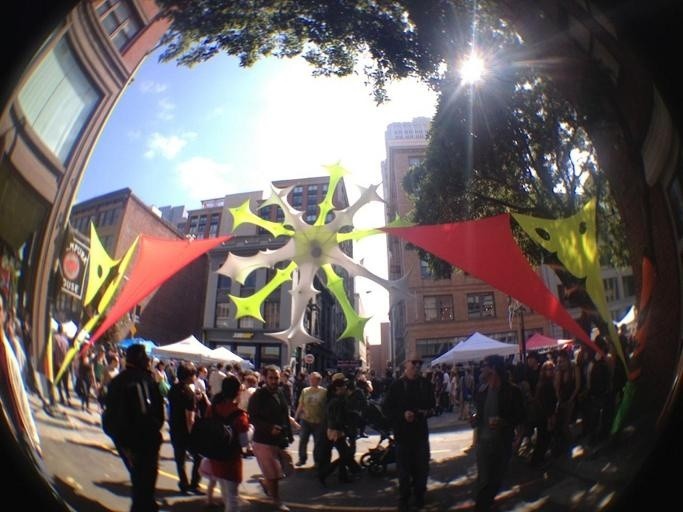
258,477 -> 273,496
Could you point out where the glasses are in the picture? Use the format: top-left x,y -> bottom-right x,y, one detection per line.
405,359 -> 424,366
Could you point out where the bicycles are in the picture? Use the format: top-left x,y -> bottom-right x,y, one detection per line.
361,430 -> 397,476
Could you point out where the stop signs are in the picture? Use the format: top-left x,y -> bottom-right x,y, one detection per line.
305,354 -> 315,364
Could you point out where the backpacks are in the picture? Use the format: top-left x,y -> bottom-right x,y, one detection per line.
189,398 -> 243,468
